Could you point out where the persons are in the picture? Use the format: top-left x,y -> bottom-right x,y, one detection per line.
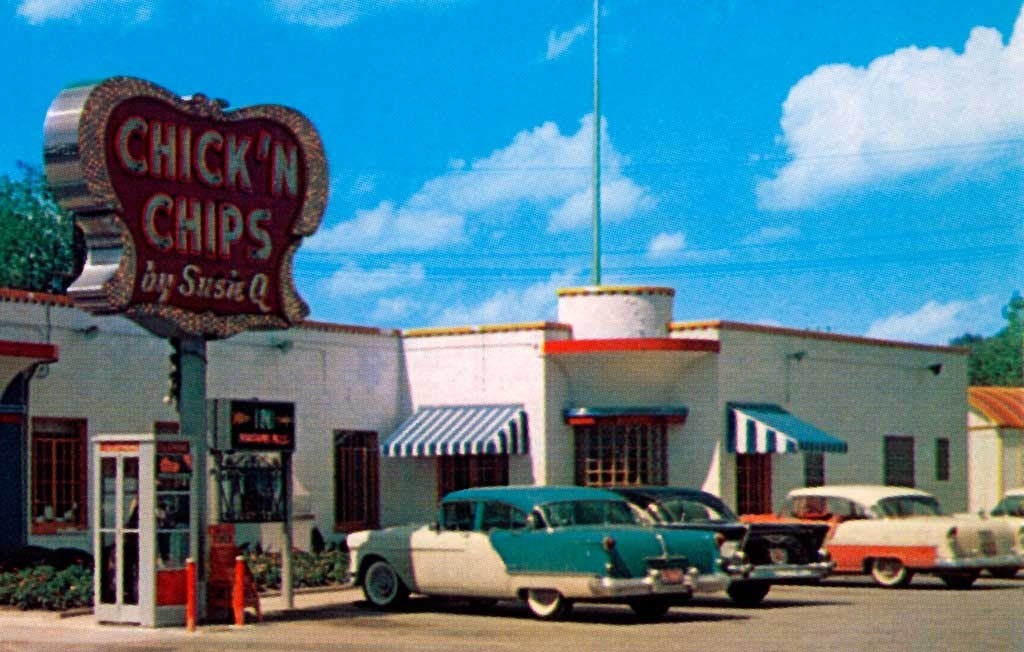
124,483 -> 164,600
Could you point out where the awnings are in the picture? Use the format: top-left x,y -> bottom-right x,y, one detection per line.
726,403 -> 847,454
380,405 -> 529,457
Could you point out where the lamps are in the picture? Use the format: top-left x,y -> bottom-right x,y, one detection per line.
927,362 -> 943,374
79,323 -> 98,337
274,339 -> 293,351
786,351 -> 806,361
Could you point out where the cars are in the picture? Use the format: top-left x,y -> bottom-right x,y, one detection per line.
346,484 -> 755,624
738,484 -> 1024,591
606,487 -> 840,605
984,490 -> 1024,579
